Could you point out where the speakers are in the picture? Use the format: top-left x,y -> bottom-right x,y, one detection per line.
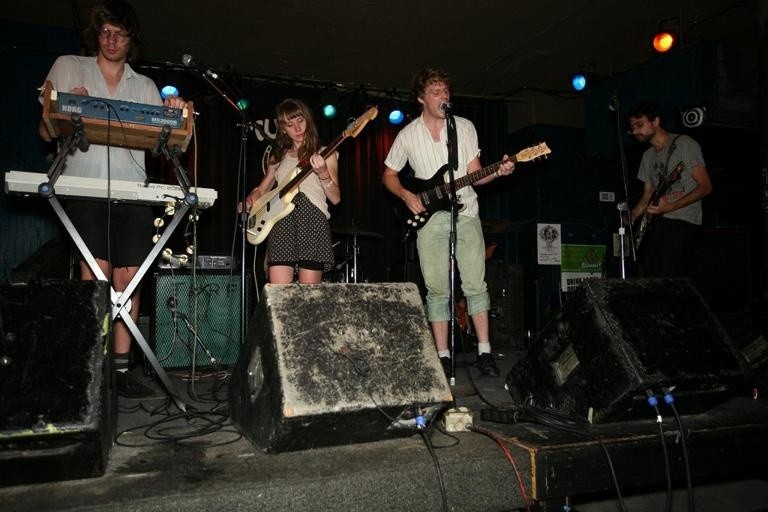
0,278 -> 119,491
226,281 -> 455,456
505,277 -> 753,424
148,269 -> 254,377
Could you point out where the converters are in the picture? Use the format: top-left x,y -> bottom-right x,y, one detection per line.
480,405 -> 521,424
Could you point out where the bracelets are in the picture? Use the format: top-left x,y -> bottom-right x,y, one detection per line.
317,175 -> 331,181
494,170 -> 501,177
322,179 -> 333,189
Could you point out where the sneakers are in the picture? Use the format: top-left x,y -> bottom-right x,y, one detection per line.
478,352 -> 500,377
440,356 -> 453,377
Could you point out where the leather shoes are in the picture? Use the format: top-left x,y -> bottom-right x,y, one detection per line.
115,370 -> 155,398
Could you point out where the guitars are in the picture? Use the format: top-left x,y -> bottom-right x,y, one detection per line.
393,142 -> 552,232
629,161 -> 683,249
245,107 -> 378,245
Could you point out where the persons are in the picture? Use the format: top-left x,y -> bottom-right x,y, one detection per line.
379,61 -> 516,380
620,99 -> 713,279
234,94 -> 342,285
29,2 -> 189,400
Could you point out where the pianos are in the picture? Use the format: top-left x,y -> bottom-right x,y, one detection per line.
4,170 -> 219,209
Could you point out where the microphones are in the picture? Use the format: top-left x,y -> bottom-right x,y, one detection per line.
167,298 -> 178,308
609,96 -> 621,111
182,53 -> 224,84
336,261 -> 346,270
440,102 -> 453,112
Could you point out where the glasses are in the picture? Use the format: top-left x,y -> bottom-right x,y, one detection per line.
626,119 -> 648,134
100,28 -> 131,43
